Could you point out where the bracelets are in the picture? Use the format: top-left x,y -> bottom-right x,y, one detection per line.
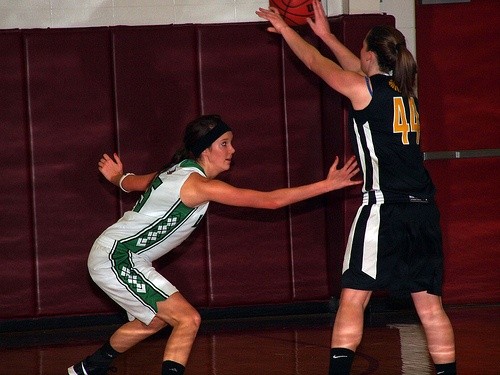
118,172 -> 137,193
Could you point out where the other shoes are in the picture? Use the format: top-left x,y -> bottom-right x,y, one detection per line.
67,361 -> 88,375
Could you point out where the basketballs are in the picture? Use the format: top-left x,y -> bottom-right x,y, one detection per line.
269,0 -> 321,28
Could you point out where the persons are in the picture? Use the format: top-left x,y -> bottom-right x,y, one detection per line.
255,0 -> 458,375
67,114 -> 363,375
